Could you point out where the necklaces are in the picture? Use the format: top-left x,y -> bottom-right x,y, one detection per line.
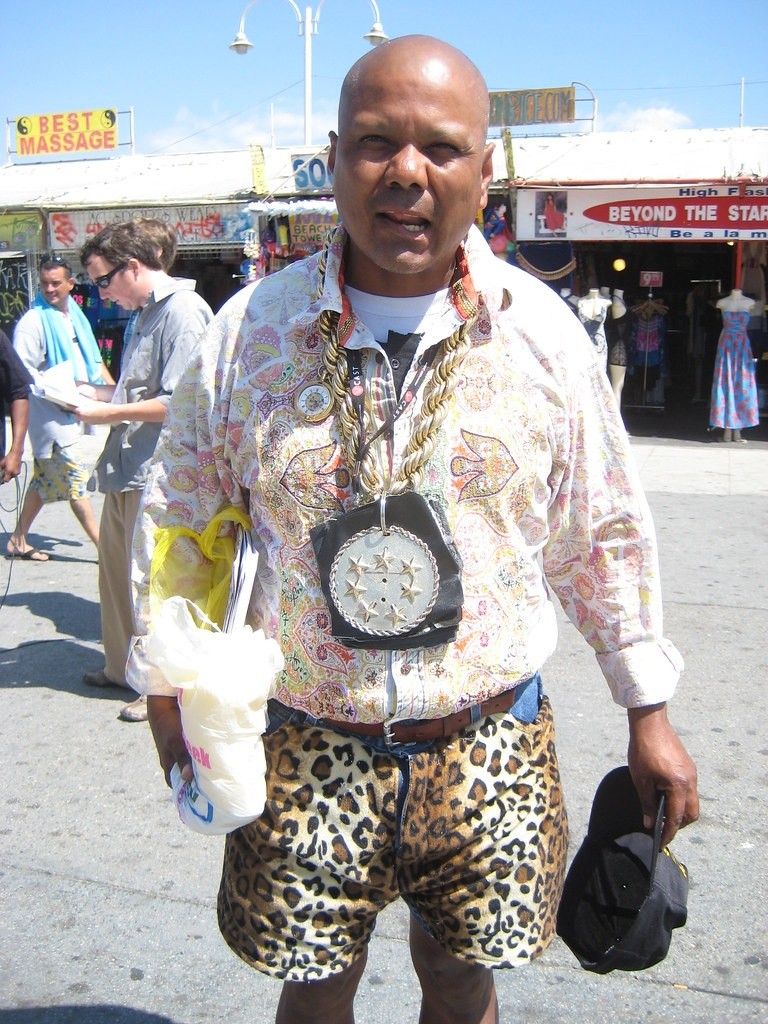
314,222 -> 479,500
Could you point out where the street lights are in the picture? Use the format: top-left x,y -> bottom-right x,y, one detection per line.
228,0 -> 392,147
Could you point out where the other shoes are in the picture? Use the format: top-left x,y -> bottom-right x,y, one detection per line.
120,695 -> 148,721
85,669 -> 116,687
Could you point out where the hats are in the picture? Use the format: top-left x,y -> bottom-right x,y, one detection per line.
556,766 -> 690,975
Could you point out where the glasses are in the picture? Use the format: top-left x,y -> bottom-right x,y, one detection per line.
41,256 -> 65,265
94,264 -> 125,289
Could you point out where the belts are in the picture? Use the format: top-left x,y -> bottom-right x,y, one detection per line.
322,688 -> 516,748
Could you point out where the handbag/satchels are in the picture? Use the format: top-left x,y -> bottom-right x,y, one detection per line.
125,507 -> 285,838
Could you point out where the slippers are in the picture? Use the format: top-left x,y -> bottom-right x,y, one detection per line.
6,546 -> 50,561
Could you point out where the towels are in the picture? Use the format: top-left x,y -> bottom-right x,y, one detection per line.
31,284 -> 112,424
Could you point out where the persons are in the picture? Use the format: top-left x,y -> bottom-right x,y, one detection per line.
555,284 -> 634,414
485,201 -> 508,241
708,288 -> 756,444
544,192 -> 565,238
0,217 -> 215,723
126,33 -> 700,1024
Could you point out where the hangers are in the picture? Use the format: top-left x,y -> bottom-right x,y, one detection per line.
629,297 -> 668,316
